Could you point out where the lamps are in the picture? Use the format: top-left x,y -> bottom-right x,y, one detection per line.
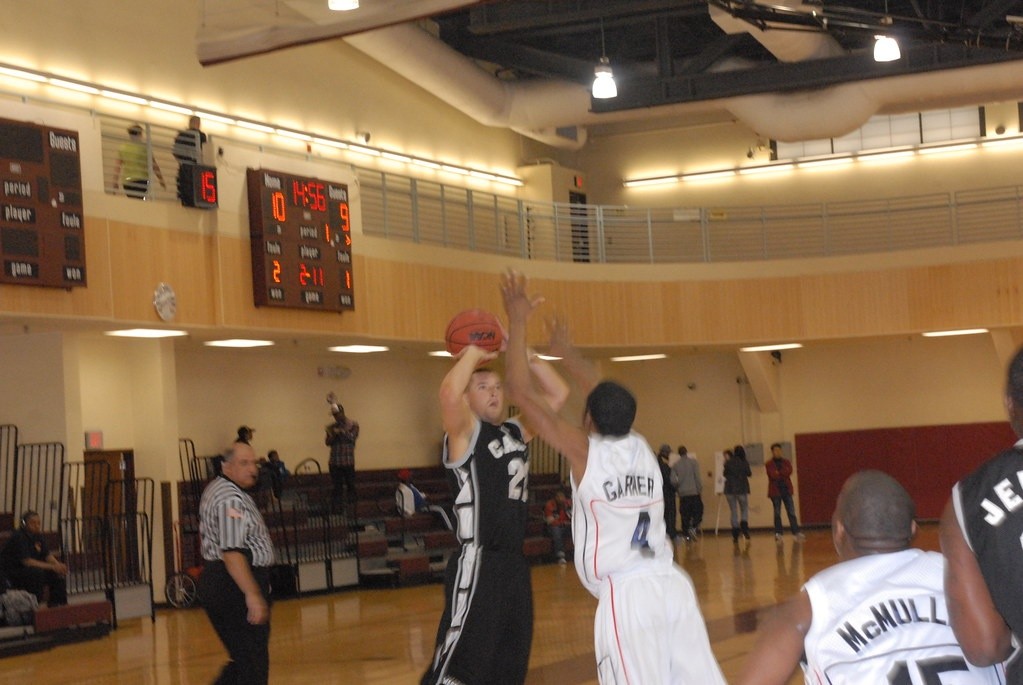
874,0 -> 900,62
591,17 -> 617,98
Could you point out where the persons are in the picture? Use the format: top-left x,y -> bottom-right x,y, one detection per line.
765,444 -> 805,540
396,471 -> 456,530
420,312 -> 570,685
173,118 -> 207,165
112,125 -> 166,199
236,426 -> 256,445
657,445 -> 703,541
498,267 -> 726,685
199,443 -> 275,685
544,491 -> 572,563
742,473 -> 1004,685
939,343 -> 1023,685
724,446 -> 750,542
0,512 -> 69,607
265,454 -> 287,498
326,405 -> 359,529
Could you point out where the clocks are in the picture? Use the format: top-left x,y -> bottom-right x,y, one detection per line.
153,282 -> 176,322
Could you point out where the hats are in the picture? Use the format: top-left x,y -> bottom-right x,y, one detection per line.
659,444 -> 672,453
399,469 -> 413,480
238,424 -> 256,436
330,402 -> 344,415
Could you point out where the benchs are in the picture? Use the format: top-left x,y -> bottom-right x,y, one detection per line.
0,512 -> 112,659
176,465 -> 573,599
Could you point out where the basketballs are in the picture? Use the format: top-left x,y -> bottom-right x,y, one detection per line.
444,309 -> 503,366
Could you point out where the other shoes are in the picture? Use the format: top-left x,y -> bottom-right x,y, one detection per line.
688,528 -> 698,540
672,536 -> 684,544
794,532 -> 807,540
555,556 -> 569,564
774,534 -> 784,542
731,528 -> 740,543
740,521 -> 750,539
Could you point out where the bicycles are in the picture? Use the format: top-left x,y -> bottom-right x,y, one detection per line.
165,566 -> 204,611
